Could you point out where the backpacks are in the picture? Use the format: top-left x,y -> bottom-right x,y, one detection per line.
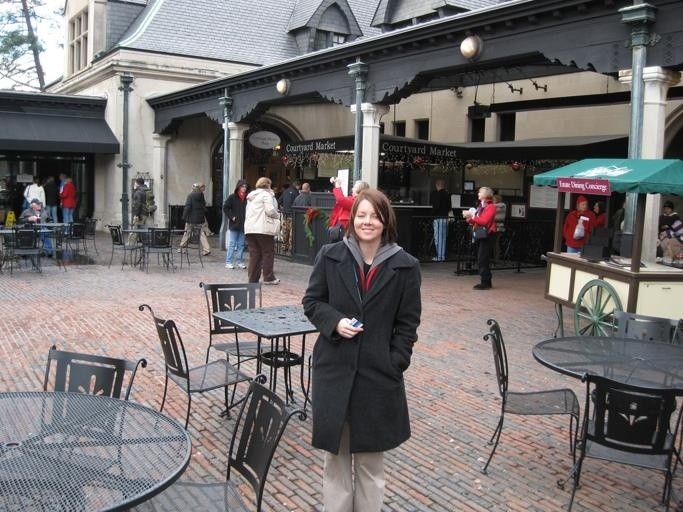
137,190 -> 156,213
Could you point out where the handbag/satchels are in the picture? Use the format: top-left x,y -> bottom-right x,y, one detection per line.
474,226 -> 488,240
328,223 -> 344,242
22,199 -> 29,209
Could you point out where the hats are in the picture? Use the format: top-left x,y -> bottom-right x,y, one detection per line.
31,198 -> 42,204
663,201 -> 672,208
575,195 -> 587,204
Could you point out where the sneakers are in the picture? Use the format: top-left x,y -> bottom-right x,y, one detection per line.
202,250 -> 210,255
225,264 -> 233,269
236,263 -> 245,269
263,278 -> 280,285
205,232 -> 214,236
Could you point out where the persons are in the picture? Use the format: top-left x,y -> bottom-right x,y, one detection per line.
302,188 -> 422,511
128,177 -> 155,248
176,178 -> 316,286
329,176 -> 369,243
20,172 -> 77,258
594,198 -> 613,257
612,200 -> 683,267
462,186 -> 507,290
430,178 -> 452,262
563,194 -> 597,253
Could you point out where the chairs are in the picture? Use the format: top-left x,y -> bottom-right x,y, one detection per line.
138,304 -> 255,457
0,346 -> 146,495
613,310 -> 671,364
199,282 -> 295,420
1,221 -> 204,276
672,319 -> 683,351
565,373 -> 682,512
481,318 -> 581,488
128,373 -> 305,512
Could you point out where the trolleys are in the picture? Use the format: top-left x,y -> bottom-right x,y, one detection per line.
531,160 -> 683,362
